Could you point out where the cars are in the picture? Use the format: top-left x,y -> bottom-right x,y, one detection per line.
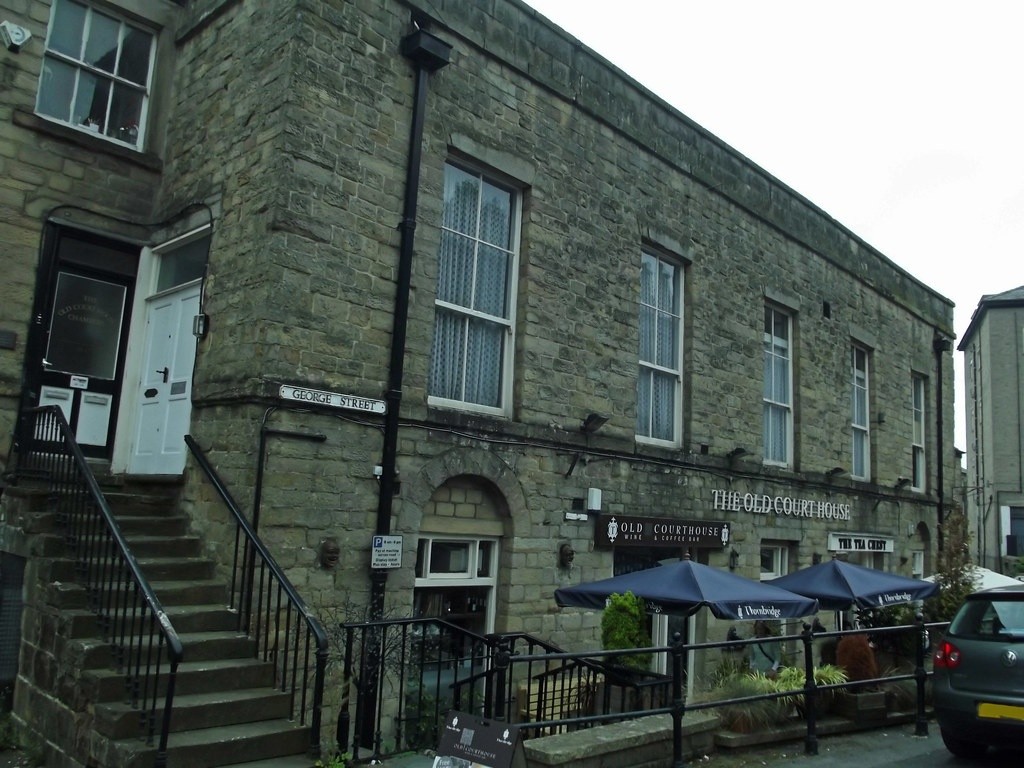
932,585 -> 1024,756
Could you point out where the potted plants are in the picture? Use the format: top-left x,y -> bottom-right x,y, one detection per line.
681,630 -> 922,734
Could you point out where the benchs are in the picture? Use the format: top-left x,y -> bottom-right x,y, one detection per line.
517,674 -> 604,739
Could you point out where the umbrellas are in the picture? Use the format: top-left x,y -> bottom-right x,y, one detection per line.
916,564 -> 1024,596
761,557 -> 938,613
554,558 -> 818,701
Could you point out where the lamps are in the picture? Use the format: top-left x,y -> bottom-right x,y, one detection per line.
728,446 -> 752,459
826,467 -> 844,476
581,412 -> 609,433
896,476 -> 910,486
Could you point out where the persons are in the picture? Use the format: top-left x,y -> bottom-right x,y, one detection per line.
835,632 -> 875,691
748,620 -> 783,681
